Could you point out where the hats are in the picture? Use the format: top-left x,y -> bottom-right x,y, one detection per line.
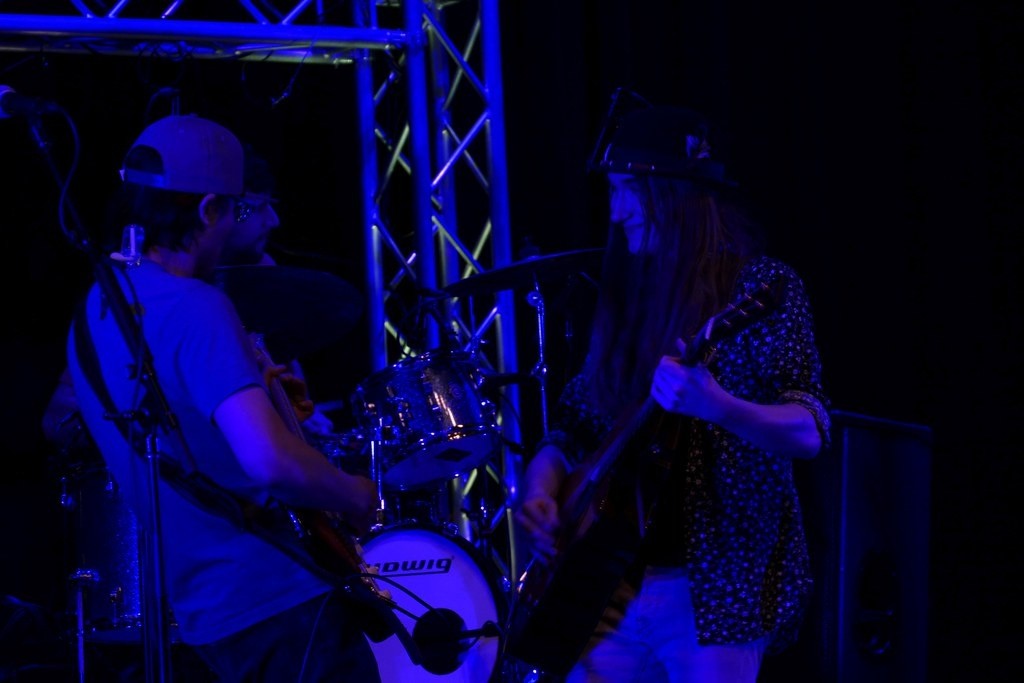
118,115 -> 253,199
587,75 -> 714,180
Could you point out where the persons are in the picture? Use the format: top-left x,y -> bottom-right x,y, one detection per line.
236,159 -> 282,269
44,111 -> 375,683
518,86 -> 835,682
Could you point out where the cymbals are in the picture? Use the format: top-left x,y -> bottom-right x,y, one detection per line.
220,263 -> 366,360
441,245 -> 601,298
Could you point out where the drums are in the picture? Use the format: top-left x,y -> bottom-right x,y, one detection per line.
348,343 -> 499,489
352,522 -> 513,683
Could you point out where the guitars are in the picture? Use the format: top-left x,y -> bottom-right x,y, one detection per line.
495,267 -> 788,679
248,331 -> 395,645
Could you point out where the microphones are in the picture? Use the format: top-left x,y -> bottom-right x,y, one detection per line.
2,84 -> 63,122
